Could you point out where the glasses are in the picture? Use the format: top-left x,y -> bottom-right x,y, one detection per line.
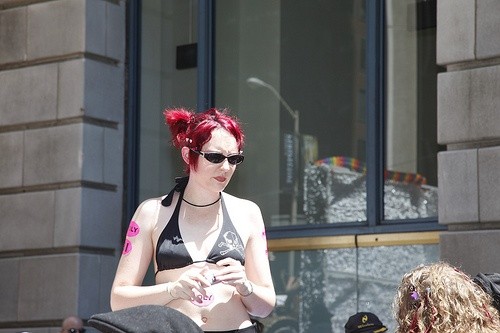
63,328 -> 86,333
188,148 -> 244,165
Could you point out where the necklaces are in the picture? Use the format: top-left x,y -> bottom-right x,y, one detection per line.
182,196 -> 221,207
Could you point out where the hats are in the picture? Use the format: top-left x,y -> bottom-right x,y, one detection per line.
86,304 -> 204,333
345,312 -> 387,333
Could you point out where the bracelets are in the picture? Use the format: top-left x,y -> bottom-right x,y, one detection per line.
236,281 -> 254,296
167,281 -> 180,301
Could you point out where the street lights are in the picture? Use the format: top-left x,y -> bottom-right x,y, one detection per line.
246,77 -> 299,276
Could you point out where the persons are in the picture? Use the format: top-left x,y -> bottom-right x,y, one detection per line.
61,316 -> 86,333
110,107 -> 277,333
392,262 -> 500,333
